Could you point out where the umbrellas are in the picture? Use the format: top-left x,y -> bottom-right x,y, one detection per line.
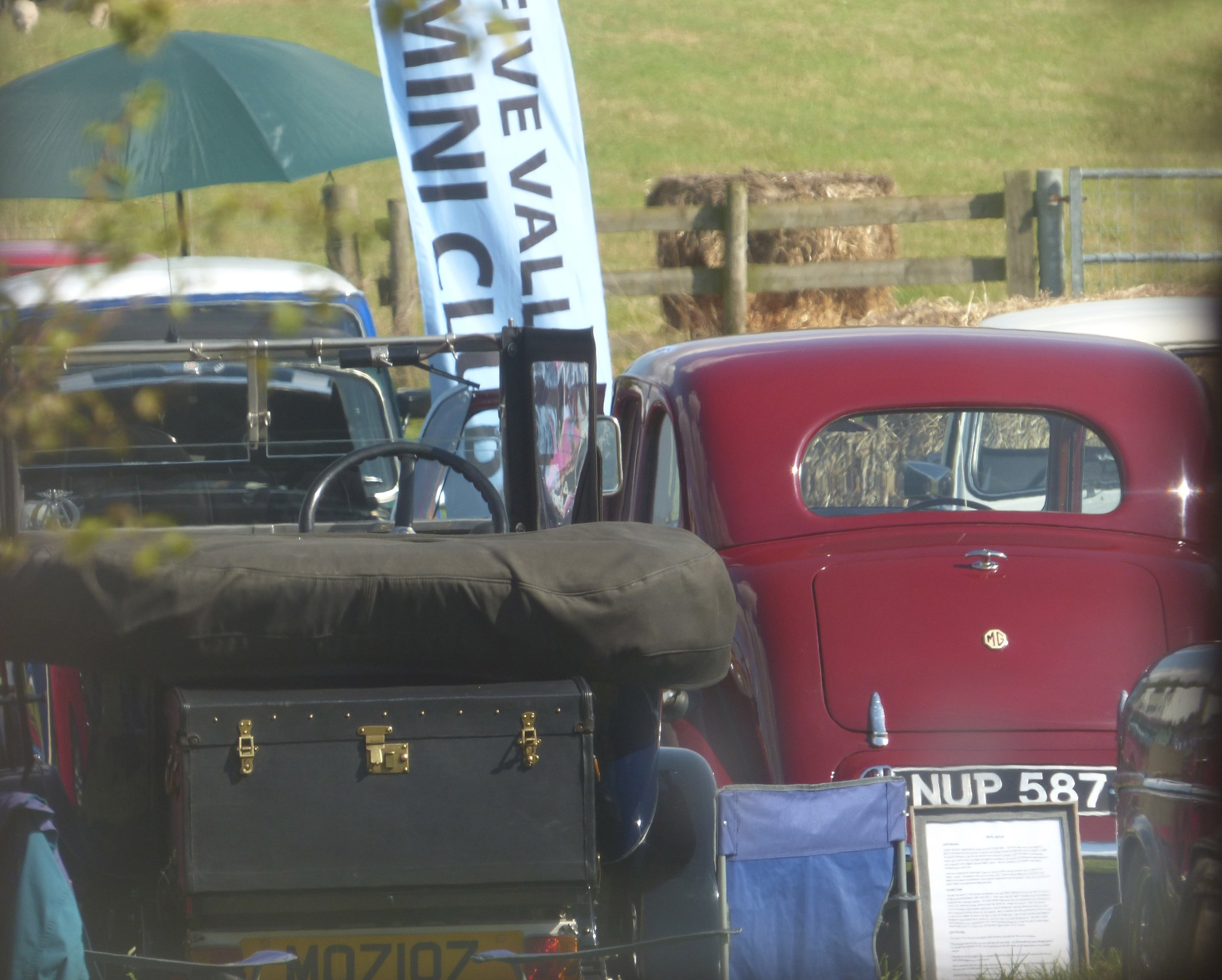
0,28 -> 396,257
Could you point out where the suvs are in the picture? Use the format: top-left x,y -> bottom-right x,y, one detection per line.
0,254 -> 453,536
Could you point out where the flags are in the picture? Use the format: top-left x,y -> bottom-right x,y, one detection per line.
369,1 -> 615,522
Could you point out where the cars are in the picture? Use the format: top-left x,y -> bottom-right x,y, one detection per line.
0,323 -> 763,980
618,327 -> 1222,980
901,295 -> 1222,524
0,237 -> 103,281
